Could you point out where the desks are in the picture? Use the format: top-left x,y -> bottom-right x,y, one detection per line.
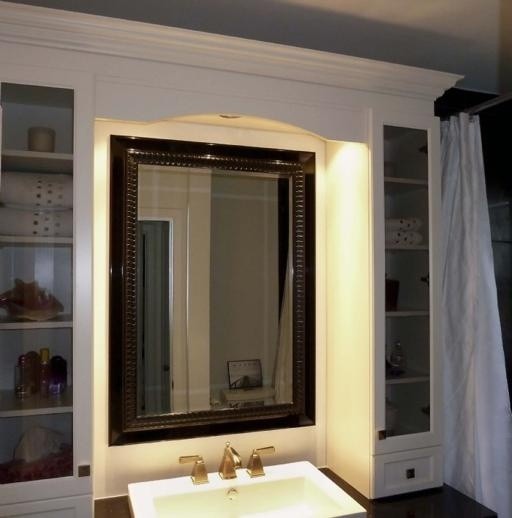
221,386 -> 275,408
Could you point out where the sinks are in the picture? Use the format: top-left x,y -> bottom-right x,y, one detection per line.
128,460 -> 368,518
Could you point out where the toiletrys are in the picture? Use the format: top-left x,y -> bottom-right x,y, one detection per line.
391,341 -> 406,368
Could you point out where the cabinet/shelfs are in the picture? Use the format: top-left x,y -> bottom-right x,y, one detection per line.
0,41 -> 95,516
330,110 -> 445,500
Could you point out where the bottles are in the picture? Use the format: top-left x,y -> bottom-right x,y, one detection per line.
14,355 -> 32,400
49,354 -> 68,395
39,347 -> 50,396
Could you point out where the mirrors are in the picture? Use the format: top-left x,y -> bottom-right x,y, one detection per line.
109,133 -> 314,447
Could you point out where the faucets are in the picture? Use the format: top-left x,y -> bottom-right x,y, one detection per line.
220,442 -> 245,480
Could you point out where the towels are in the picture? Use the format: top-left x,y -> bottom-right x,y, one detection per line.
385,215 -> 423,245
1,169 -> 73,236
